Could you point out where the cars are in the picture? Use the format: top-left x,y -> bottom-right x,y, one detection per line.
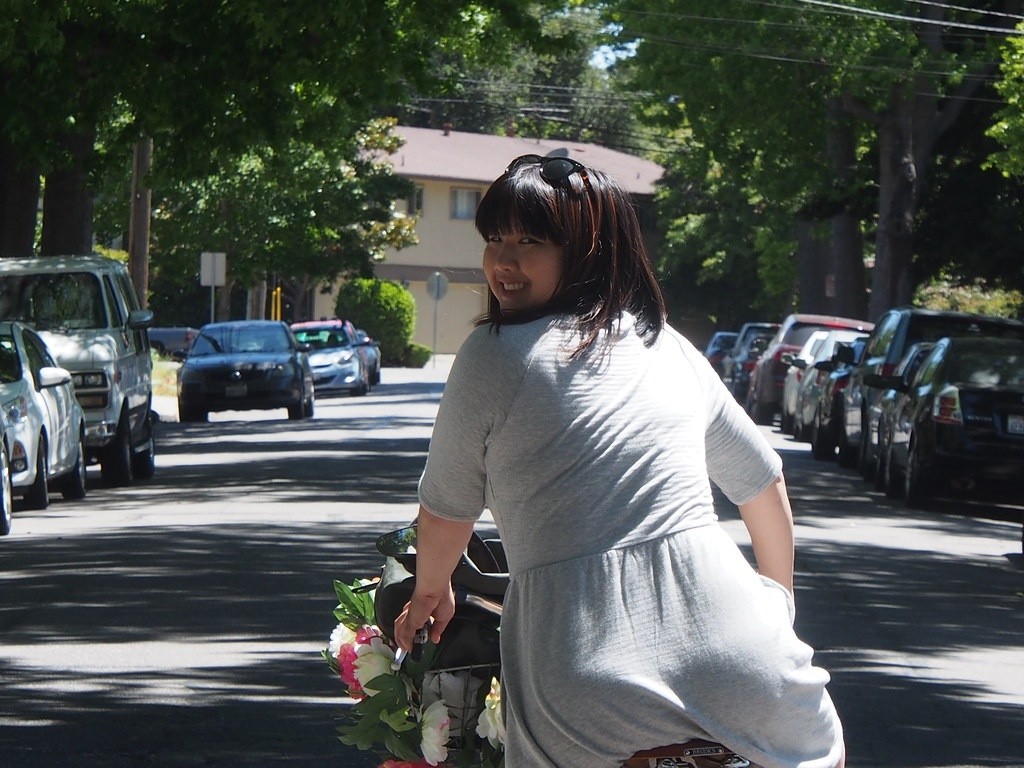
864,336 -> 1024,510
357,329 -> 382,386
171,320 -> 316,422
0,321 -> 87,508
707,315 -> 877,459
288,318 -> 369,397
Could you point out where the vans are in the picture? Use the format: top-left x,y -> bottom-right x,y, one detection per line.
0,253 -> 158,483
840,307 -> 1024,494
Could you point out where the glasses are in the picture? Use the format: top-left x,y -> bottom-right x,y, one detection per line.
504,154 -> 599,216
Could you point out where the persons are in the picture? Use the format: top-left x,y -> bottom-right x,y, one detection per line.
327,334 -> 339,346
34,275 -> 83,331
392,164 -> 847,768
0,348 -> 18,383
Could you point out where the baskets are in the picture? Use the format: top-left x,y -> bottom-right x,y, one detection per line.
407,661 -> 499,752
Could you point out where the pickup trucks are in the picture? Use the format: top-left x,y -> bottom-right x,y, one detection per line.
148,327 -> 200,353
